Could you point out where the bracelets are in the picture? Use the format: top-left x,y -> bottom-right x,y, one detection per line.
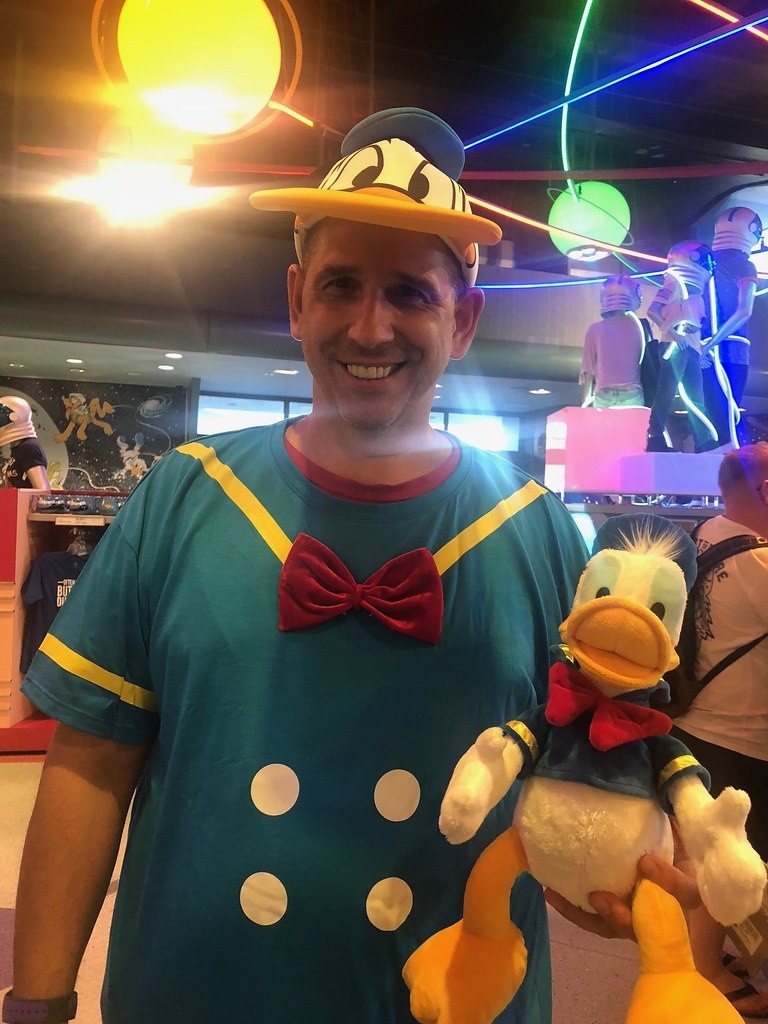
2,988 -> 78,1024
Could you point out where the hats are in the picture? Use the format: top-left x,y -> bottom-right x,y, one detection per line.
251,107 -> 503,286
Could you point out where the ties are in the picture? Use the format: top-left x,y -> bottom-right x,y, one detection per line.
277,533 -> 445,644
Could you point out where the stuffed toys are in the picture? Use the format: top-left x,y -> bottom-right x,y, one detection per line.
400,513 -> 767,1024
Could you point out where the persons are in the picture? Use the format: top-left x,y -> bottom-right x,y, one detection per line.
578,275 -> 646,408
0,395 -> 52,489
668,446 -> 768,1019
647,239 -> 734,453
703,207 -> 764,447
2,105 -> 702,1024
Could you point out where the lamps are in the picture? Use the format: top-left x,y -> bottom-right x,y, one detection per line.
40,105 -> 238,234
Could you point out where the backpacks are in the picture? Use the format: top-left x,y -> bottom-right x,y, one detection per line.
653,519 -> 767,720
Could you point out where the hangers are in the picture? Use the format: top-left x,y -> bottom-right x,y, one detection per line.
66,528 -> 94,556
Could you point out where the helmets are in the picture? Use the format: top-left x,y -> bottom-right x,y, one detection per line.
0,396 -> 37,446
